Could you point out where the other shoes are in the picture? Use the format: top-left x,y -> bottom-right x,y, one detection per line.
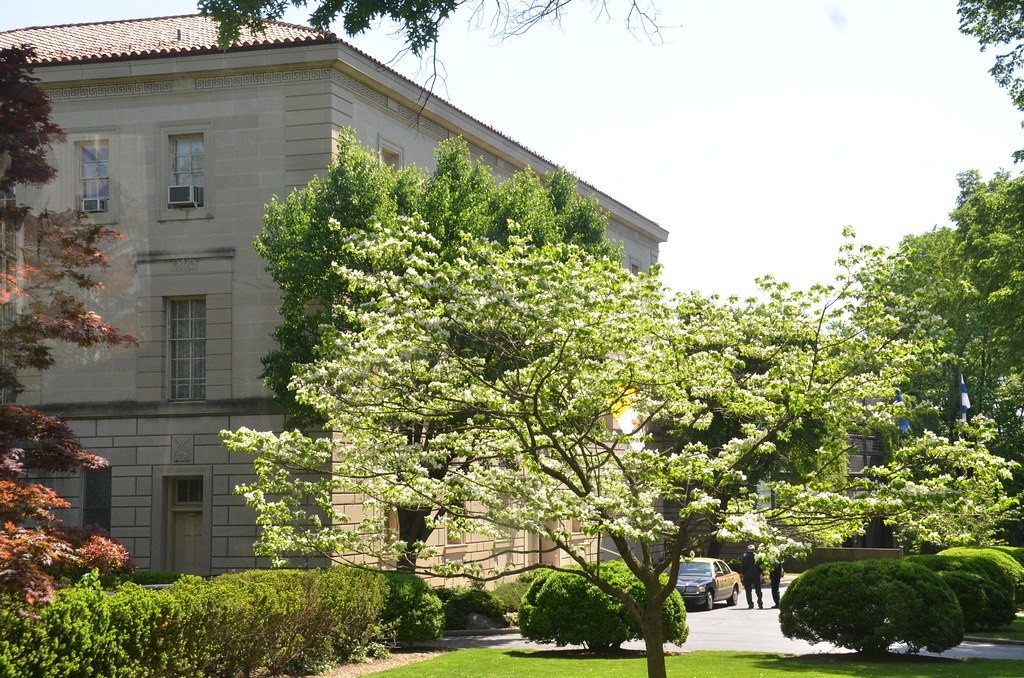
772,605 -> 780,608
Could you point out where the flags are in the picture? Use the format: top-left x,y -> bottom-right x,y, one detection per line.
897,383 -> 909,432
960,374 -> 972,413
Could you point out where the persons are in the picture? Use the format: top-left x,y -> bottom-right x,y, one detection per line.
741,545 -> 764,609
769,558 -> 782,608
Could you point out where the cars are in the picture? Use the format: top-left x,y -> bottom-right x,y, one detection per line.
663,556 -> 743,610
